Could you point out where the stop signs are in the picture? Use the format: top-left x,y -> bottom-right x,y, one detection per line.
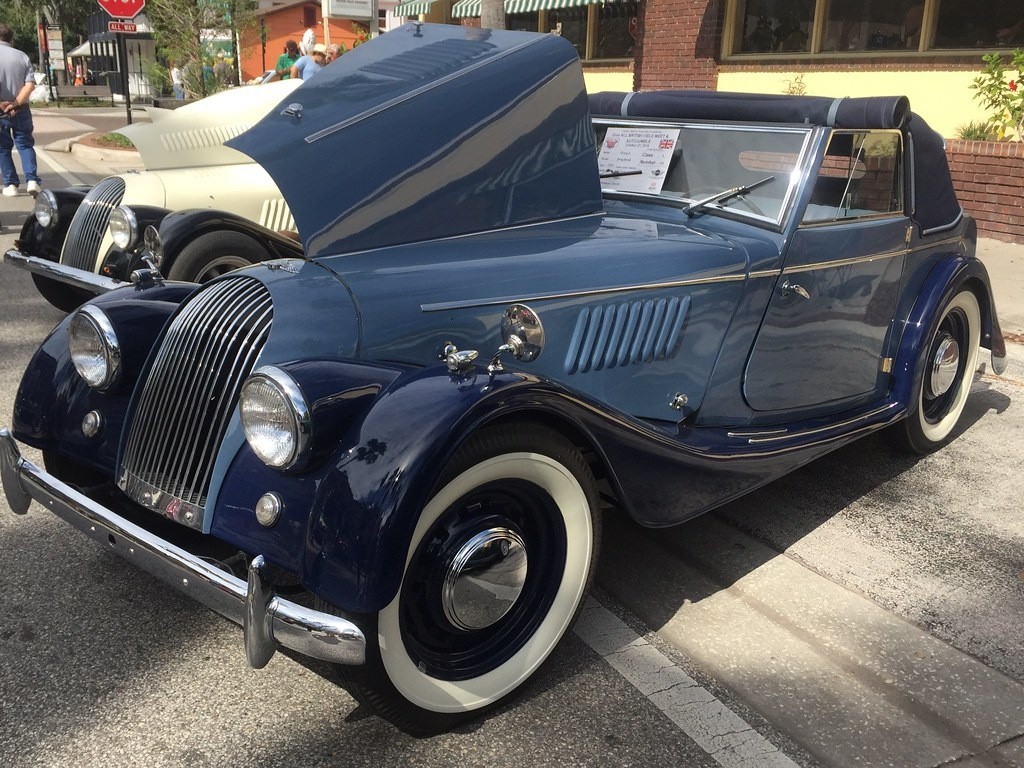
96,0 -> 146,20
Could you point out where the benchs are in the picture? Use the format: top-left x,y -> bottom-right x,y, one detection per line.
55,84 -> 115,107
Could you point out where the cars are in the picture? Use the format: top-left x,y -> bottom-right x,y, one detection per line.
1,20 -> 1008,734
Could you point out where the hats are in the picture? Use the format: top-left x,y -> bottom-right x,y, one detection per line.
313,44 -> 328,56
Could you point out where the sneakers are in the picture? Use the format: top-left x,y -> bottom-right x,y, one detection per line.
27,180 -> 41,199
2,184 -> 19,196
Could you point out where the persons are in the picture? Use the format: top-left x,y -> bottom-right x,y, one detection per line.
0,25 -> 41,199
170,53 -> 234,100
276,40 -> 339,82
75,74 -> 98,101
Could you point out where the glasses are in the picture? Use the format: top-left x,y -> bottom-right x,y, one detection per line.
288,47 -> 296,51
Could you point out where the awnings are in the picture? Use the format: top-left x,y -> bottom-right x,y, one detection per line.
452,0 -> 607,19
393,0 -> 437,17
